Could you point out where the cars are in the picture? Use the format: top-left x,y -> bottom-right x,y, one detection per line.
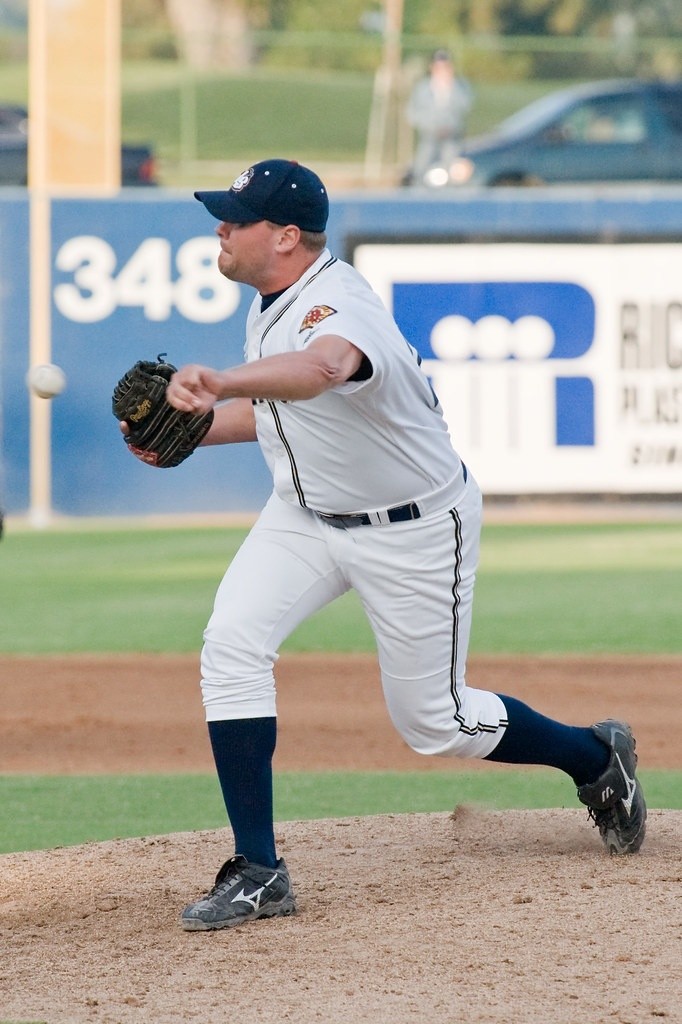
396,71 -> 682,193
0,100 -> 160,188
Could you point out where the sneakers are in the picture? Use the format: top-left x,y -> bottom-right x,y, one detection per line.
576,719 -> 647,855
182,854 -> 295,930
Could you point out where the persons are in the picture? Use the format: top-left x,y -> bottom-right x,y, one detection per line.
110,159 -> 647,930
121,145 -> 158,186
404,49 -> 476,185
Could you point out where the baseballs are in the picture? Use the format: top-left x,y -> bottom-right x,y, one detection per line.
29,364 -> 68,399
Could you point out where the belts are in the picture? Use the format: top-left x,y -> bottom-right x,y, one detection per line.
315,502 -> 420,529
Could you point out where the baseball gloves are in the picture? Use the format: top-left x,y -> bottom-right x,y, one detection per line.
110,359 -> 216,469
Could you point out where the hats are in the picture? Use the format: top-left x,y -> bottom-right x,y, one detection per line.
194,159 -> 329,232
